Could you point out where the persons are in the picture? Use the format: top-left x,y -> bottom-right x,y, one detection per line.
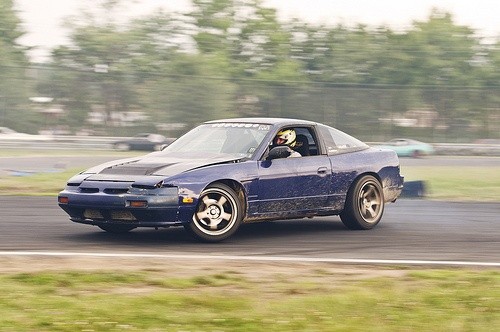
269,129 -> 303,159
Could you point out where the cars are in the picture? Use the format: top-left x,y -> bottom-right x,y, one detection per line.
365,138 -> 434,159
112,133 -> 178,153
58,117 -> 406,240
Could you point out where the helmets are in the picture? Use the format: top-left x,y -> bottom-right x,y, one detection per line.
274,128 -> 296,147
